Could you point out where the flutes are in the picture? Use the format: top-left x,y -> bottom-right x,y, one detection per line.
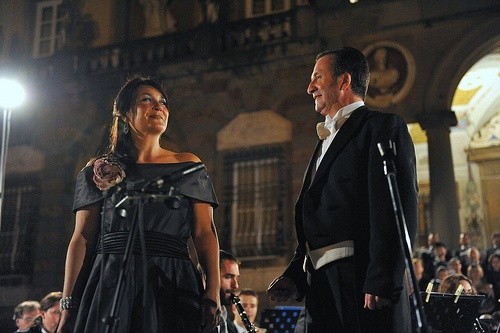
230,295 -> 256,333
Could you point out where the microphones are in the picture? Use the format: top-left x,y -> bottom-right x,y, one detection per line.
142,162 -> 205,193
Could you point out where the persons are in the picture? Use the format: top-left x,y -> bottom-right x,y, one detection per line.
56,78 -> 220,333
197,250 -> 267,333
405,232 -> 500,333
12,291 -> 63,333
265,47 -> 419,333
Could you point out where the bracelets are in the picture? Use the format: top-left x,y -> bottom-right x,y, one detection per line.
60,297 -> 80,311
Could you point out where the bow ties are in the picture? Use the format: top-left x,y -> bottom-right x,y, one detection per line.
315,118 -> 338,141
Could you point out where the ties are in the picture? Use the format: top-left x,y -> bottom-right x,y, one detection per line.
220,315 -> 226,333
